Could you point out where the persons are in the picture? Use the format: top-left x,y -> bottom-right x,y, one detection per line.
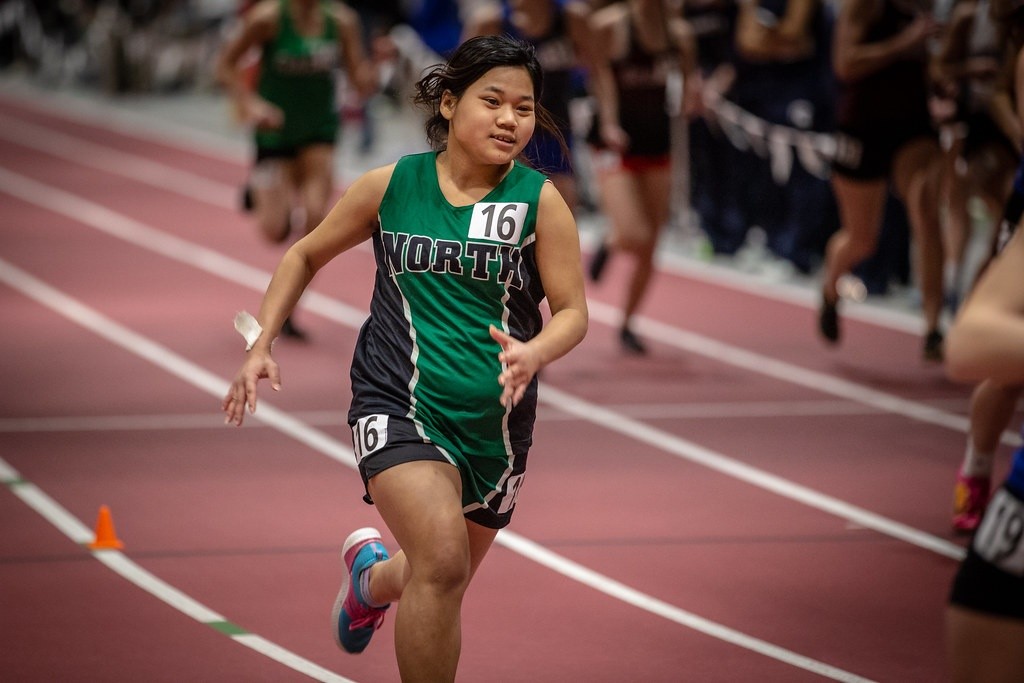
216,1 -> 1023,371
926,375 -> 1024,679
219,31 -> 588,682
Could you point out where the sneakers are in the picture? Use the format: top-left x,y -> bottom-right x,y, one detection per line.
819,301 -> 838,340
924,329 -> 943,362
954,469 -> 992,533
329,527 -> 391,654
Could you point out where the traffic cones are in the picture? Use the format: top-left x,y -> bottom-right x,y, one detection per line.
87,503 -> 124,550
